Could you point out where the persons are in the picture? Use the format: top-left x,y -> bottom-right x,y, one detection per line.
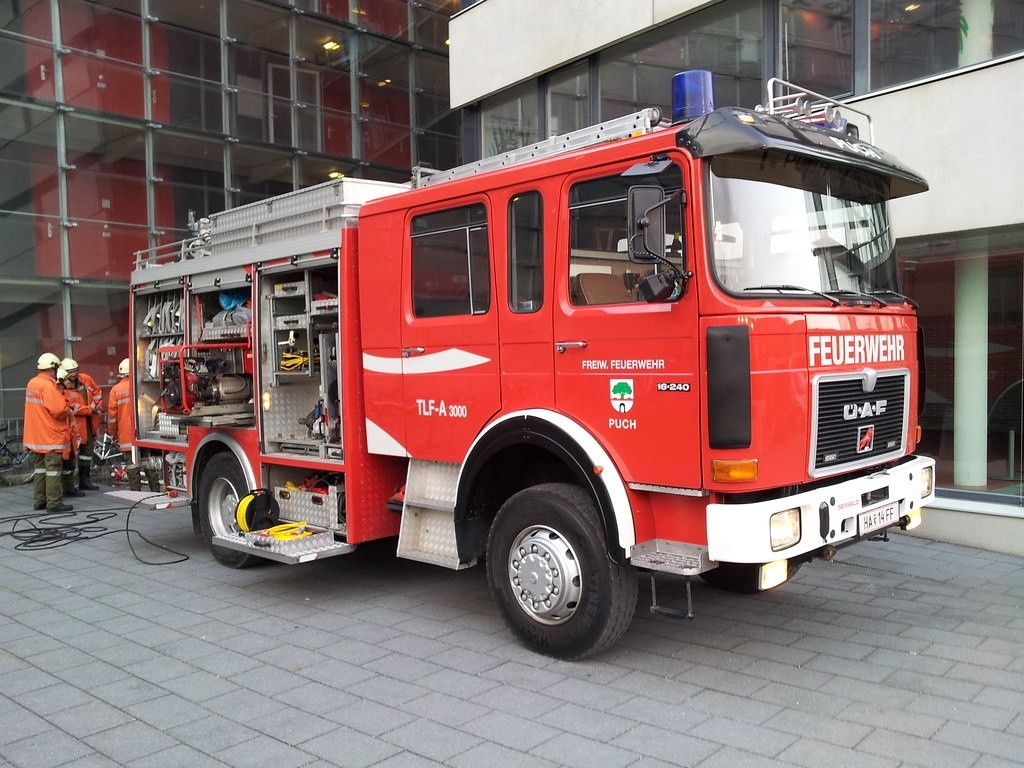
107,358 -> 161,492
56,358 -> 102,496
22,353 -> 73,513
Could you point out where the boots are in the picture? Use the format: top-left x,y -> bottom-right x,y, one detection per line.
62,475 -> 86,497
79,465 -> 99,490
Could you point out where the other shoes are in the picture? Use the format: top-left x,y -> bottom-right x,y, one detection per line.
46,504 -> 73,513
34,504 -> 46,511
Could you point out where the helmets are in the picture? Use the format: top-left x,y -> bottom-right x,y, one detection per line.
55,370 -> 70,383
61,358 -> 79,372
36,353 -> 61,369
119,358 -> 130,374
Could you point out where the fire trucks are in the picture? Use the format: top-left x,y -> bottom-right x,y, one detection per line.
104,70 -> 937,661
